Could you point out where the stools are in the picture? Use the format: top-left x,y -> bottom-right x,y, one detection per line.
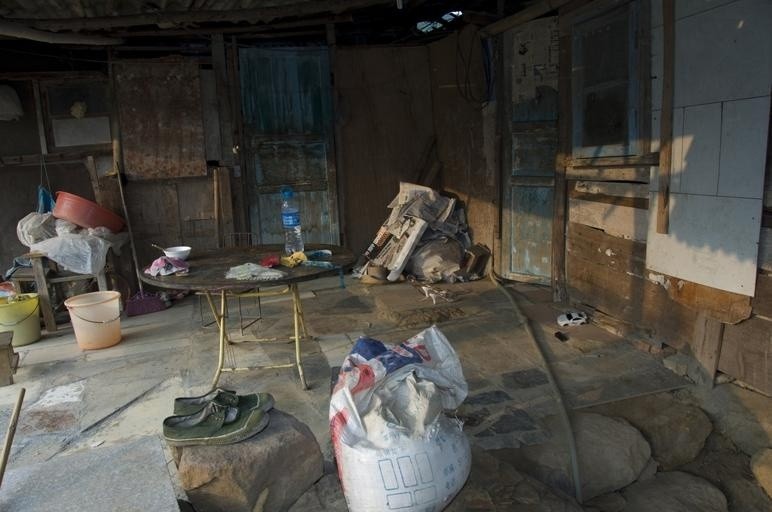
8,266 -> 52,295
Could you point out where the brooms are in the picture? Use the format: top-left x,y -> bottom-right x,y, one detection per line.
114,161 -> 166,317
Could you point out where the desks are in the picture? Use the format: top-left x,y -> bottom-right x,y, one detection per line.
137,243 -> 359,392
21,245 -> 125,339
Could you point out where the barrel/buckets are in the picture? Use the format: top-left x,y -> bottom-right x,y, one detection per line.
0,292 -> 42,348
63,290 -> 123,350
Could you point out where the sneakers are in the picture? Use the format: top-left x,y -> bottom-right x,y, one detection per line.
163,387 -> 274,415
162,400 -> 270,447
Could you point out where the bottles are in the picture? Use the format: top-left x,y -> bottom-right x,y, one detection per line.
280,191 -> 305,255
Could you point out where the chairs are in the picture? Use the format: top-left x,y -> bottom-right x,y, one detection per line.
193,232 -> 262,337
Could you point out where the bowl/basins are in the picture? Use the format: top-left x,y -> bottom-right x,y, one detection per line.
164,246 -> 192,261
51,190 -> 125,235
